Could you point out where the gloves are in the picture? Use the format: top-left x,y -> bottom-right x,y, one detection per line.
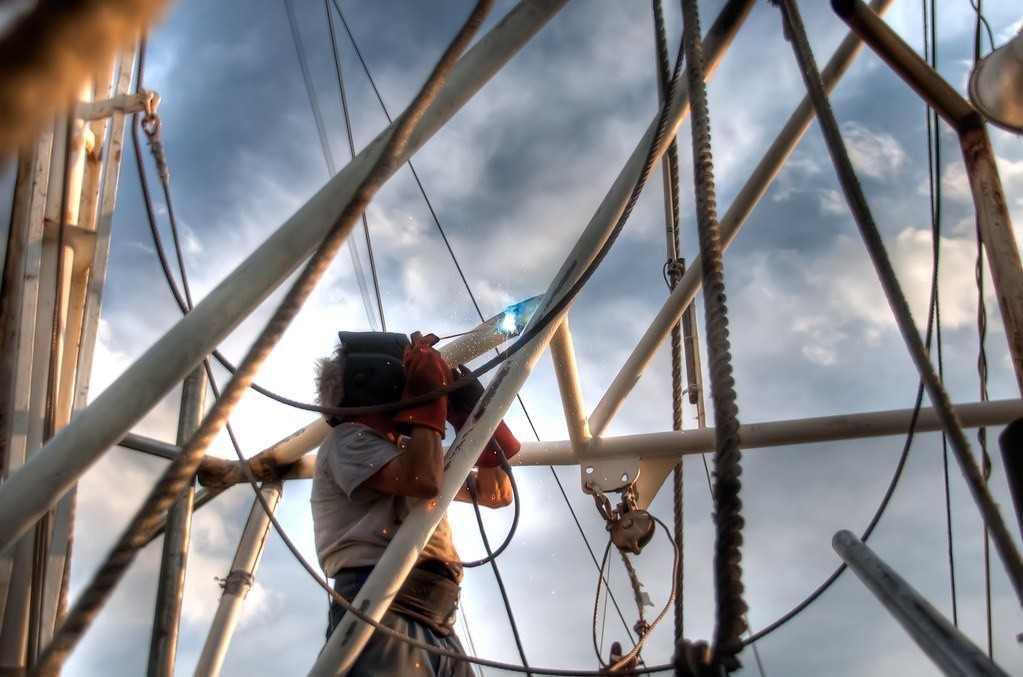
445,363 -> 523,468
394,332 -> 455,438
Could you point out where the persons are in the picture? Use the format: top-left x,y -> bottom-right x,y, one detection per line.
309,332 -> 521,676
